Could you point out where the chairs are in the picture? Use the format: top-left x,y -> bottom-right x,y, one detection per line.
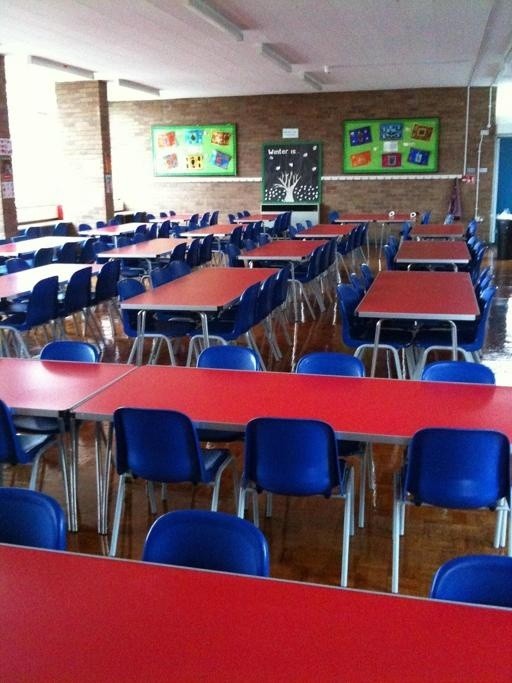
175,211 -> 220,231
464,219 -> 499,294
0,357 -> 140,474
39,339 -> 97,362
258,272 -> 293,358
0,275 -> 68,358
58,265 -> 103,354
11,338 -> 115,474
336,283 -> 412,376
294,352 -> 374,528
142,508 -> 271,577
429,554 -> 512,608
393,426 -> 512,592
224,219 -> 263,245
266,212 -> 292,233
0,542 -> 512,682
202,233 -> 214,263
151,241 -> 187,268
90,260 -> 120,336
288,245 -> 324,320
186,237 -> 201,268
185,280 -> 261,370
237,415 -> 355,587
150,267 -> 201,328
0,487 -> 67,552
351,218 -> 414,285
109,405 -> 239,556
229,210 -> 252,223
415,285 -> 499,380
275,266 -> 293,348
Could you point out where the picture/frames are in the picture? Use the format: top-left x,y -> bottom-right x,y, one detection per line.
263,141 -> 323,205
341,115 -> 441,173
151,122 -> 236,176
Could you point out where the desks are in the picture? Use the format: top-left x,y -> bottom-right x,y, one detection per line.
238,239 -> 329,321
408,223 -> 466,241
0,262 -> 105,336
148,211 -> 204,222
0,234 -> 89,261
233,213 -> 278,224
353,270 -> 481,377
76,222 -> 153,247
295,223 -> 359,239
116,266 -> 282,365
180,223 -> 249,250
69,363 -> 512,535
96,238 -> 194,275
394,239 -> 472,271
0,357 -> 139,531
335,212 -> 417,257
